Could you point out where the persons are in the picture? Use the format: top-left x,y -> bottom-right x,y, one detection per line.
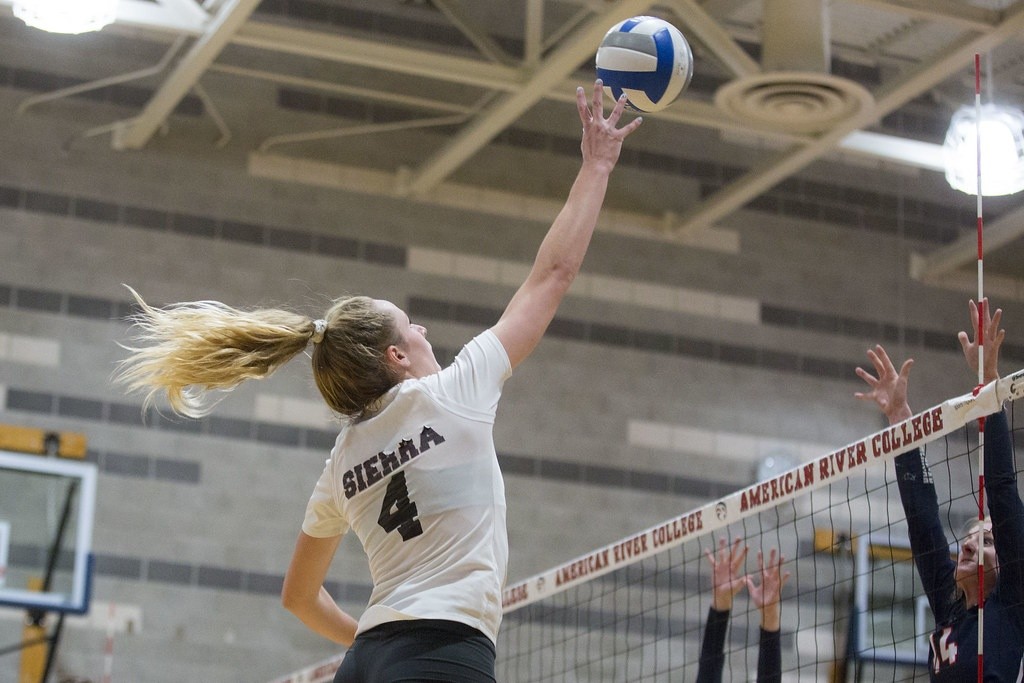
854,297 -> 1024,683
116,78 -> 644,683
695,537 -> 790,683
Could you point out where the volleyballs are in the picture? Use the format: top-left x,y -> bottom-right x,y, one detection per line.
593,14 -> 694,114
